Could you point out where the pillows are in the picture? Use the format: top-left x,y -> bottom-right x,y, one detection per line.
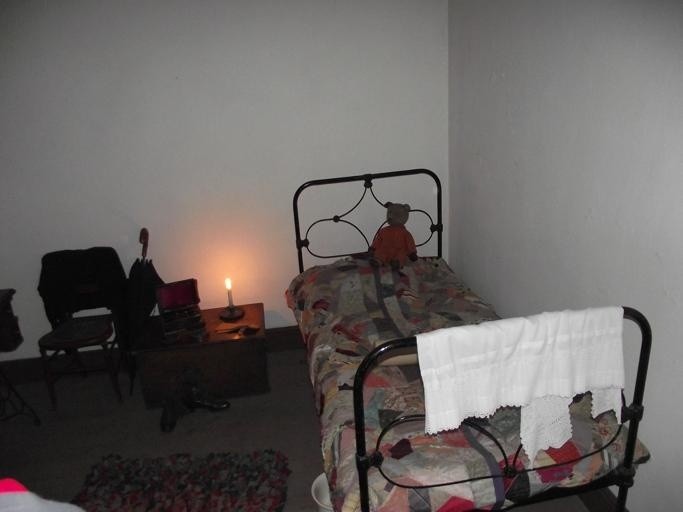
285,264 -> 330,311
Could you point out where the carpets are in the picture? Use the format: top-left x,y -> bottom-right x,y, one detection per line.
71,450 -> 292,512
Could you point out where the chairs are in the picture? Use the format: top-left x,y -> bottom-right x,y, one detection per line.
39,245 -> 127,410
0,288 -> 40,427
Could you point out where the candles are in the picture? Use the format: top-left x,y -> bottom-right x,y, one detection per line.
224,277 -> 234,309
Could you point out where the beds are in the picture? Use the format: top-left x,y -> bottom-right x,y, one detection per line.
285,168 -> 654,511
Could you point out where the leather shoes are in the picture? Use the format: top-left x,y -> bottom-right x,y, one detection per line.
160,398 -> 179,432
186,392 -> 230,412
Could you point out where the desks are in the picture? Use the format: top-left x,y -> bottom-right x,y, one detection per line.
130,301 -> 272,410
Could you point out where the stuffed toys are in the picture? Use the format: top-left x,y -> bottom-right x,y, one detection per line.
358,200 -> 420,271
